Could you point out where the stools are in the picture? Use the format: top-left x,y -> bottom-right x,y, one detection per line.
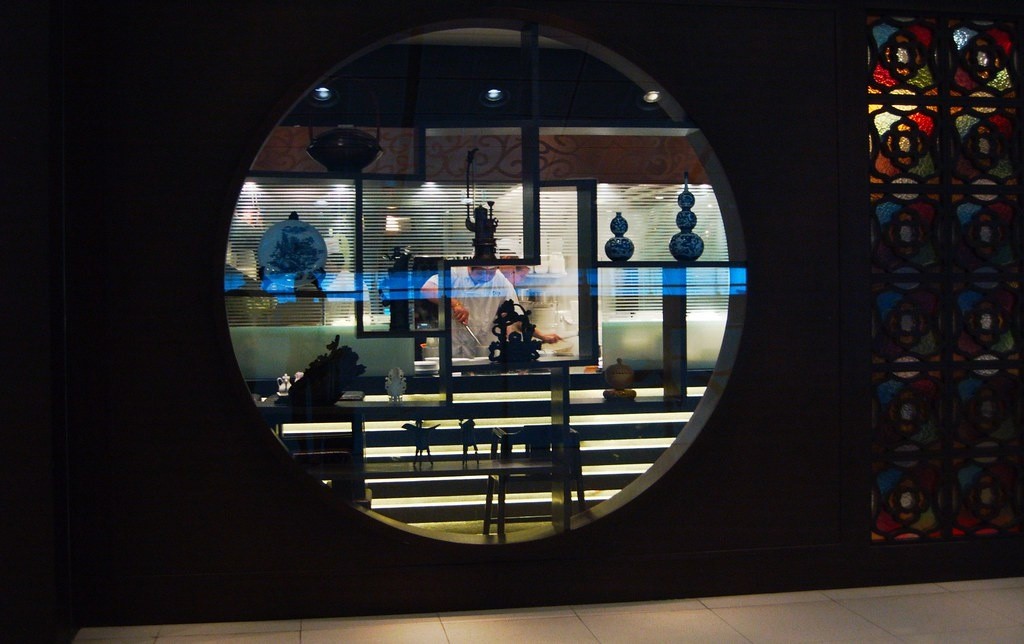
482,425 -> 586,536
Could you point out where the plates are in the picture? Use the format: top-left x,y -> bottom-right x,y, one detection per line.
554,352 -> 575,358
257,219 -> 329,289
452,357 -> 489,366
413,370 -> 440,375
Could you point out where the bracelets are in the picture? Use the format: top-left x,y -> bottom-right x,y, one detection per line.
451,304 -> 460,307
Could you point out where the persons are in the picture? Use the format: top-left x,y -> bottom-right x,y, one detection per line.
420,238 -> 563,358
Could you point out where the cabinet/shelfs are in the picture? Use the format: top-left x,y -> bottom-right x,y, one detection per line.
224,26 -> 746,544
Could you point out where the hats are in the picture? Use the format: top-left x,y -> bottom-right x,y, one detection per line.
470,244 -> 500,270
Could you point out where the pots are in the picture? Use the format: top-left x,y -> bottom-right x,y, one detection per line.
470,345 -> 493,356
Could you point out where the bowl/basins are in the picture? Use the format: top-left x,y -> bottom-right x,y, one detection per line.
413,357 -> 440,369
603,358 -> 637,391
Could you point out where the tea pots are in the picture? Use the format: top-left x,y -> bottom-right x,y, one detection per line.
276,373 -> 292,394
